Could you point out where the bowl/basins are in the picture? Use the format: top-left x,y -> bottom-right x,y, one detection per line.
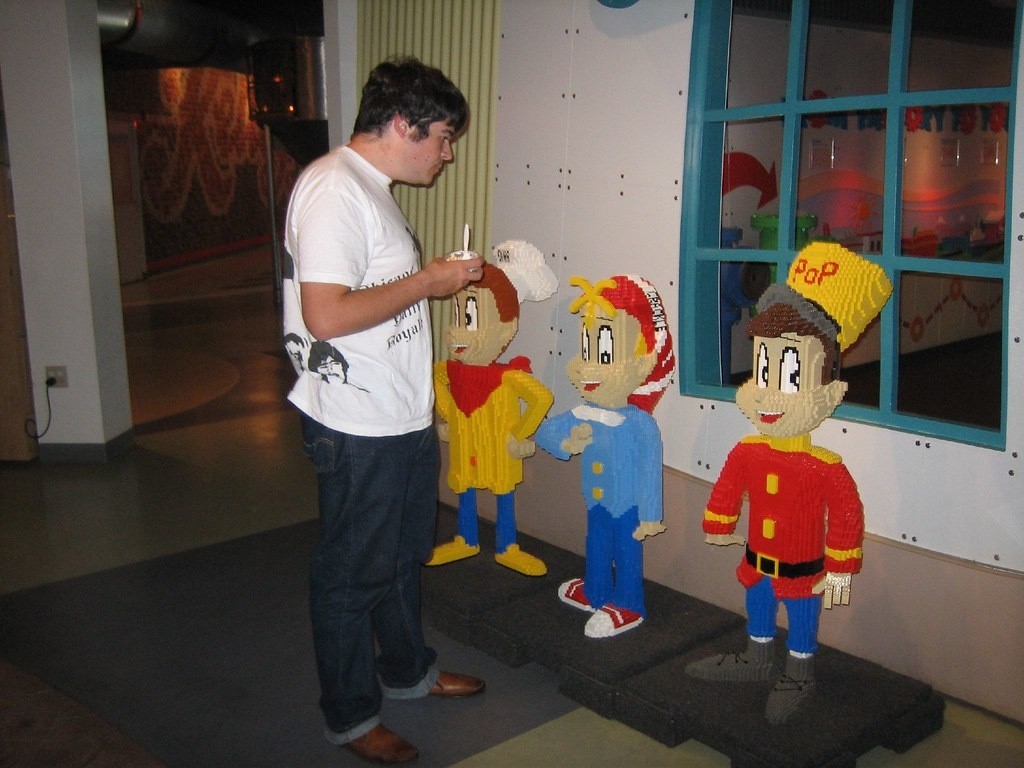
444,250 -> 480,272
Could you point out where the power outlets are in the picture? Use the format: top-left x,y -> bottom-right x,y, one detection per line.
45,365 -> 69,388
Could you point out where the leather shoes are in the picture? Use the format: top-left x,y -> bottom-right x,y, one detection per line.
429,669 -> 485,696
340,723 -> 420,768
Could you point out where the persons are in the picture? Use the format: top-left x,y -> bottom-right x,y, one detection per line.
422,240 -> 558,575
275,54 -> 486,764
533,273 -> 675,640
684,242 -> 894,728
